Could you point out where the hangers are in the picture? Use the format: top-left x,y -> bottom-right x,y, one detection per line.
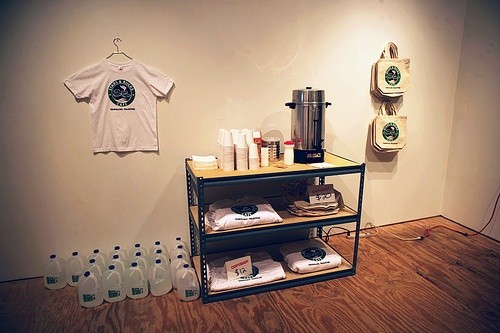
107,36 -> 131,61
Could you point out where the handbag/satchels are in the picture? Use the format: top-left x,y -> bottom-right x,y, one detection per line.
370,42 -> 410,153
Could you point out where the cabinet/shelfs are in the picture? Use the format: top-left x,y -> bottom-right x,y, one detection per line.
185,152 -> 365,304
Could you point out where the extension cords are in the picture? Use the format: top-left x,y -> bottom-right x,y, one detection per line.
346,233 -> 370,238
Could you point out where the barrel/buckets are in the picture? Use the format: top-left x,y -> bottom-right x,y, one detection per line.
285,87 -> 331,164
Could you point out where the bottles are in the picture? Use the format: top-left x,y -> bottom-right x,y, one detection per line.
123,262 -> 149,299
284,141 -> 295,165
83,259 -> 102,276
149,249 -> 170,265
87,249 -> 106,266
64,252 -> 84,286
107,255 -> 126,272
44,255 -> 67,289
78,271 -> 103,308
176,264 -> 200,301
100,265 -> 126,303
128,244 -> 147,256
126,252 -> 148,269
170,245 -> 191,261
148,259 -> 172,296
170,255 -> 191,288
149,241 -> 167,254
171,237 -> 188,249
108,246 -> 126,260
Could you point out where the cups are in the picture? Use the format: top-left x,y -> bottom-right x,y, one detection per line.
217,128 -> 280,172
